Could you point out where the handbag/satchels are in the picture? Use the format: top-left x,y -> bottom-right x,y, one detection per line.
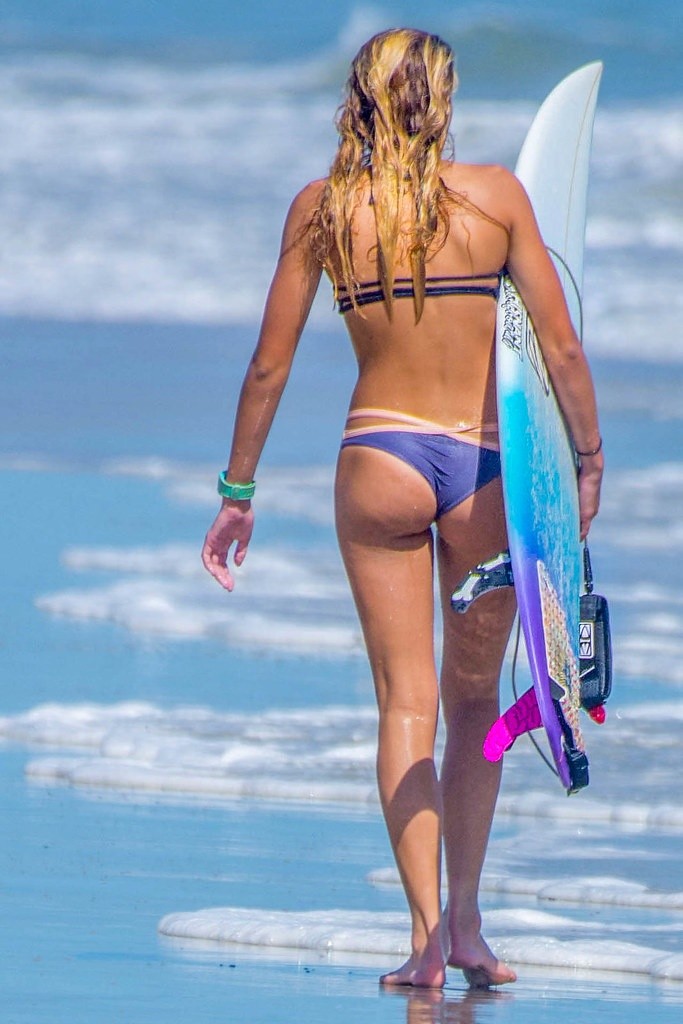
579,594 -> 611,710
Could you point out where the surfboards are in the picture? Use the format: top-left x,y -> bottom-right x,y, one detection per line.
488,61 -> 605,795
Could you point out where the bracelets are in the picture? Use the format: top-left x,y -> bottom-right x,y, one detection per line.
219,471 -> 255,500
575,438 -> 602,456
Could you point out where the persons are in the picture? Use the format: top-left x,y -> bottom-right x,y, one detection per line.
202,27 -> 604,988
